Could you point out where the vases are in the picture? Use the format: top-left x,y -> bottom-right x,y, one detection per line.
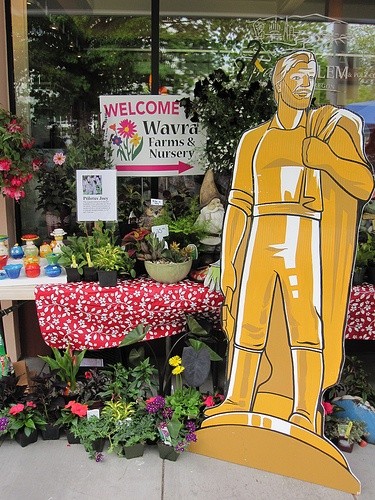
85,437 -> 105,452
156,439 -> 180,461
66,431 -> 81,443
0,228 -> 67,279
15,429 -> 37,447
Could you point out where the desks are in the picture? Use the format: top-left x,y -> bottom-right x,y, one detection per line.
35,273 -> 375,383
0,256 -> 68,363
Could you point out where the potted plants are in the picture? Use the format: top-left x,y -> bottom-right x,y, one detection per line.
359,430 -> 369,447
78,233 -> 99,282
112,416 -> 155,458
353,243 -> 374,284
137,232 -> 192,284
38,340 -> 87,405
61,236 -> 82,282
33,369 -> 63,440
334,417 -> 355,453
99,243 -> 136,287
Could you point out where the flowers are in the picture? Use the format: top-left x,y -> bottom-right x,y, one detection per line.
77,415 -> 108,464
0,102 -> 64,200
322,401 -> 344,436
146,355 -> 200,452
0,401 -> 48,437
54,401 -> 88,435
123,228 -> 180,258
